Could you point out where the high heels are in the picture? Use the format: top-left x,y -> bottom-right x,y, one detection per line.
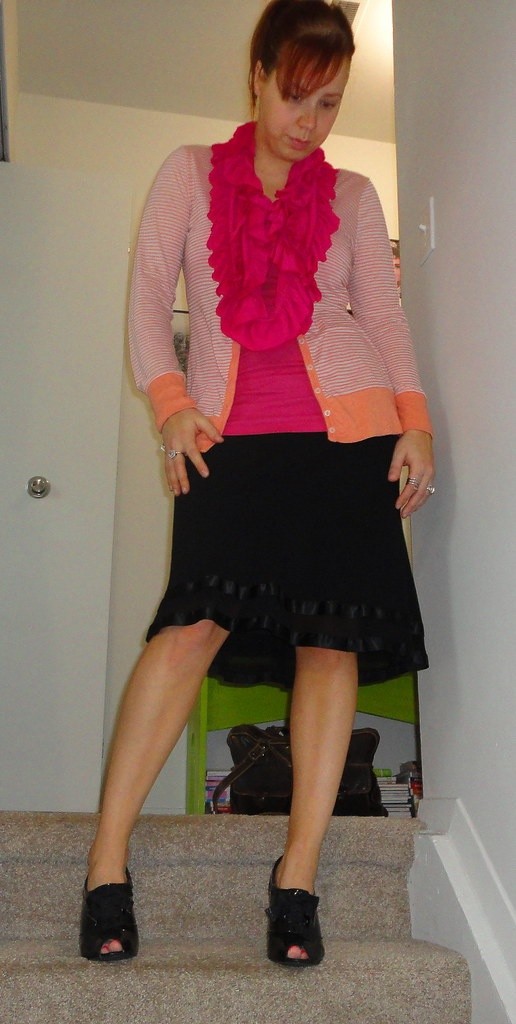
264,854 -> 327,969
79,867 -> 139,961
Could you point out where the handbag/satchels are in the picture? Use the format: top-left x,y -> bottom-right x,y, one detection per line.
210,722 -> 390,817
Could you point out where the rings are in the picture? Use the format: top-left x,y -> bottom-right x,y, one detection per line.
167,449 -> 184,458
406,477 -> 420,488
427,484 -> 435,494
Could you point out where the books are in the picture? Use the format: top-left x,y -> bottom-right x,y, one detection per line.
204,770 -> 231,812
373,760 -> 423,818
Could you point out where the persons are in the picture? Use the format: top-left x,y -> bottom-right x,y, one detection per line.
76,0 -> 432,968
